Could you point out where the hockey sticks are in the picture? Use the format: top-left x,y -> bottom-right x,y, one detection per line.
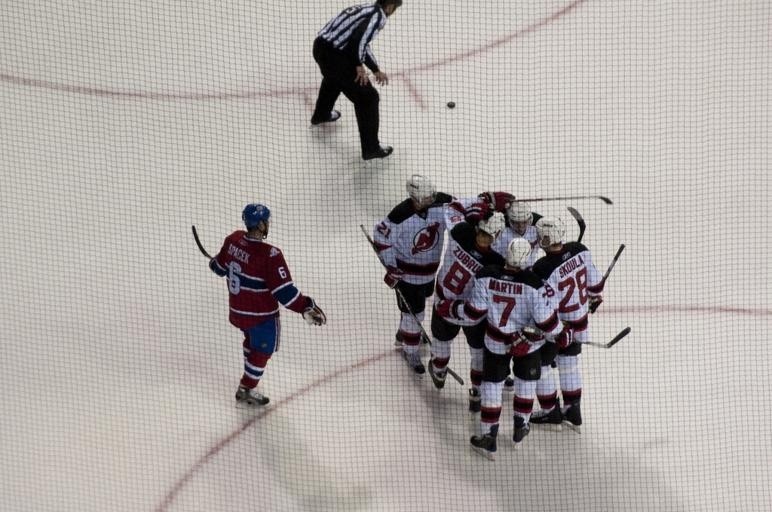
361,225 -> 464,386
572,328 -> 630,348
192,226 -> 214,260
512,195 -> 613,204
567,207 -> 586,243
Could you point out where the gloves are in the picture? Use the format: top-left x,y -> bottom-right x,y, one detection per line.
437,299 -> 464,320
554,325 -> 574,350
303,299 -> 326,326
384,265 -> 404,289
587,294 -> 602,313
464,191 -> 513,225
505,328 -> 532,357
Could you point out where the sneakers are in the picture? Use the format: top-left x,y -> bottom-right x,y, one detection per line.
470,375 -> 582,426
362,146 -> 392,159
236,386 -> 270,405
311,110 -> 341,124
395,329 -> 448,389
513,415 -> 529,442
471,424 -> 499,451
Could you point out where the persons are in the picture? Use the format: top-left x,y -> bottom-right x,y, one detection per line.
209,204 -> 326,405
311,0 -> 402,160
374,175 -> 602,451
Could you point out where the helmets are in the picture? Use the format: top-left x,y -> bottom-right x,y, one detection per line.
478,198 -> 566,268
242,204 -> 269,229
406,175 -> 437,207
